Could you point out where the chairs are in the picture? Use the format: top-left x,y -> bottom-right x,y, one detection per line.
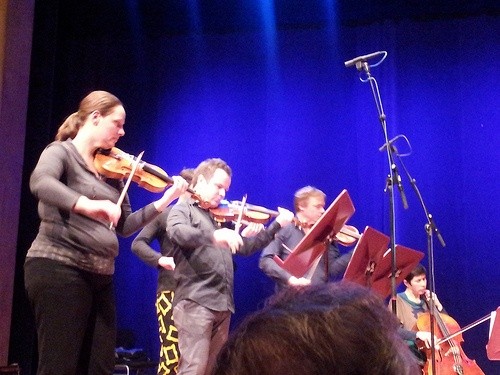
114,328 -> 158,375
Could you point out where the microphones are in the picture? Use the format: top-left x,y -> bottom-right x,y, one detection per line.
379,135 -> 401,153
344,51 -> 383,68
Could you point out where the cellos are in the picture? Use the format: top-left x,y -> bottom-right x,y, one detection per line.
413,292 -> 485,375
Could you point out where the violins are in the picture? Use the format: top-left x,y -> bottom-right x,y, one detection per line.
94,147 -> 205,208
209,199 -> 307,230
333,224 -> 362,247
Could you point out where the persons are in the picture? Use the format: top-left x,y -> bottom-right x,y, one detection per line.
24,90 -> 190,375
130,167 -> 265,374
210,282 -> 424,374
258,186 -> 363,294
387,262 -> 450,375
167,158 -> 295,375
